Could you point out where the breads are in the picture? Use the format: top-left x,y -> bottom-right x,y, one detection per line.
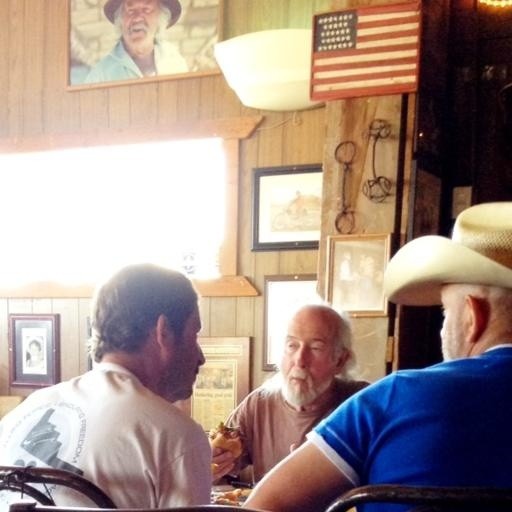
210,487 -> 253,506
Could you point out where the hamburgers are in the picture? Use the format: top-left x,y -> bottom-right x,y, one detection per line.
210,421 -> 244,462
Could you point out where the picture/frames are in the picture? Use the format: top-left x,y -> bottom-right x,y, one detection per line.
174,336 -> 251,456
250,163 -> 391,374
5,312 -> 64,395
65,2 -> 228,94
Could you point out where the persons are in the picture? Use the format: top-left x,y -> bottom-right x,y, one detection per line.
85,0 -> 187,85
208,303 -> 373,488
25,341 -> 46,375
242,200 -> 512,510
0,263 -> 214,511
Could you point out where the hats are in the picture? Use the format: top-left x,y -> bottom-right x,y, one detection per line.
104,0 -> 182,28
381,199 -> 512,308
